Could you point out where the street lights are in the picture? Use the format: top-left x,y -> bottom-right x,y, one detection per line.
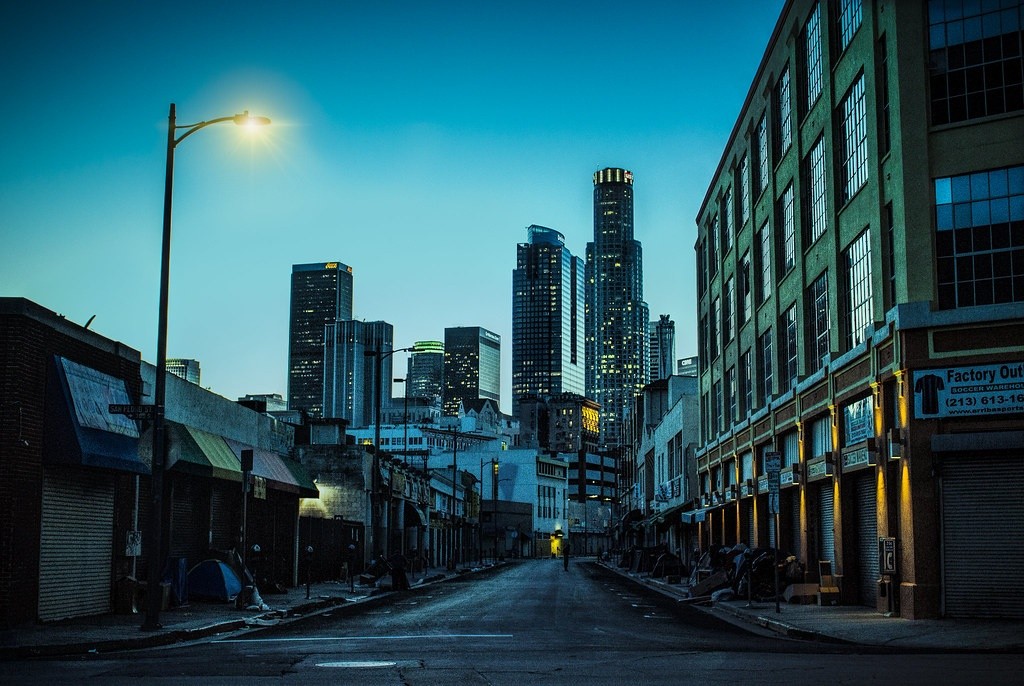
139,101 -> 278,630
368,343 -> 416,589
451,430 -> 478,576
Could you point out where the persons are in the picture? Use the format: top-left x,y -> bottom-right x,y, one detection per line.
452,542 -> 496,568
384,551 -> 410,590
573,540 -> 605,563
563,541 -> 570,572
